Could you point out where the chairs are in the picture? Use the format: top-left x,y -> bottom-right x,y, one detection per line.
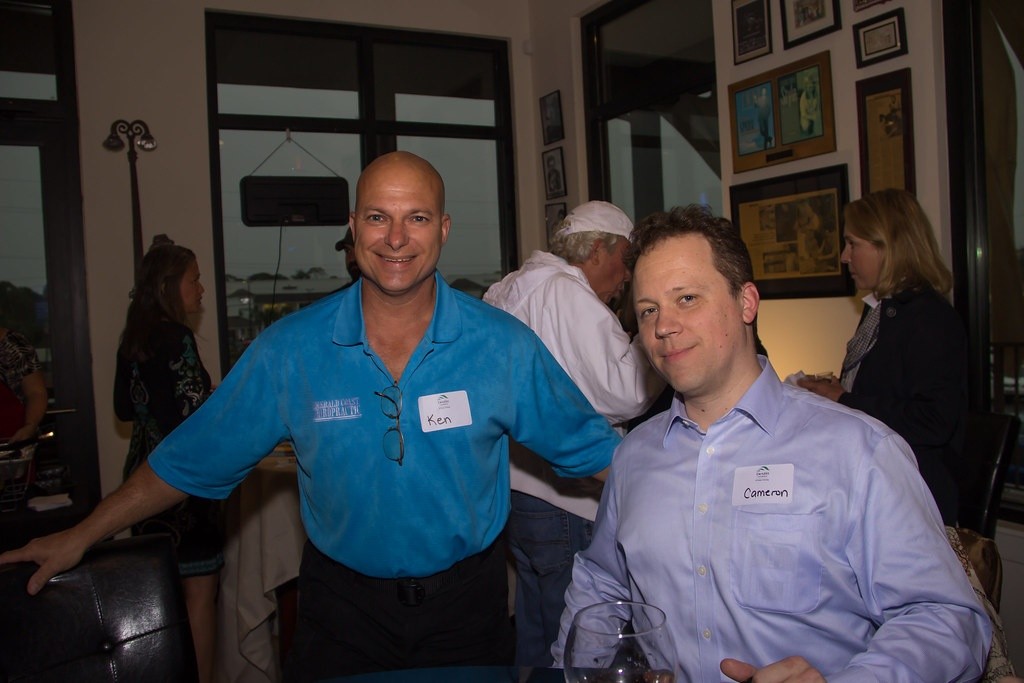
0,534 -> 199,683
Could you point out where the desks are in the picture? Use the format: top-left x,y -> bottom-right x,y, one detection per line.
313,665 -> 629,683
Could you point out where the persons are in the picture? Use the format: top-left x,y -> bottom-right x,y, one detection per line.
799,76 -> 818,135
113,245 -> 225,683
796,197 -> 837,267
330,232 -> 362,293
0,151 -> 623,683
0,326 -> 49,480
798,187 -> 1004,610
482,199 -> 666,667
550,205 -> 995,683
753,86 -> 773,150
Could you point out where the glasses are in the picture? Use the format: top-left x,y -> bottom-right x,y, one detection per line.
374,381 -> 404,467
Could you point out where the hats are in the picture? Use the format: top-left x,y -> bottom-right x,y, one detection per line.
335,226 -> 356,252
556,201 -> 634,243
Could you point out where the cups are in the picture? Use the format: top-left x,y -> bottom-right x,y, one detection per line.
563,601 -> 678,683
803,372 -> 832,385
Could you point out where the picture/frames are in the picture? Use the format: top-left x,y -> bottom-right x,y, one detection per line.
731,0 -> 773,65
854,68 -> 916,200
545,202 -> 567,247
540,89 -> 565,145
732,166 -> 856,301
852,8 -> 908,69
727,49 -> 836,173
779,0 -> 842,49
542,147 -> 567,200
